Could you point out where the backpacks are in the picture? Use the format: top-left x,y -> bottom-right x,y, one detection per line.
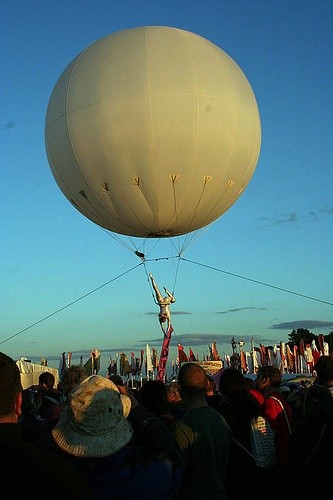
232,412 -> 277,475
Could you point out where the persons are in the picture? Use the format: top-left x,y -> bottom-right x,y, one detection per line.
0,351 -> 333,500
148,272 -> 175,338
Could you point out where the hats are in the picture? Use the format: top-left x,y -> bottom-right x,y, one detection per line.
49,375 -> 134,458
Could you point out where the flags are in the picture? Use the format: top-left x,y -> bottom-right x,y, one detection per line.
22,334 -> 330,380
